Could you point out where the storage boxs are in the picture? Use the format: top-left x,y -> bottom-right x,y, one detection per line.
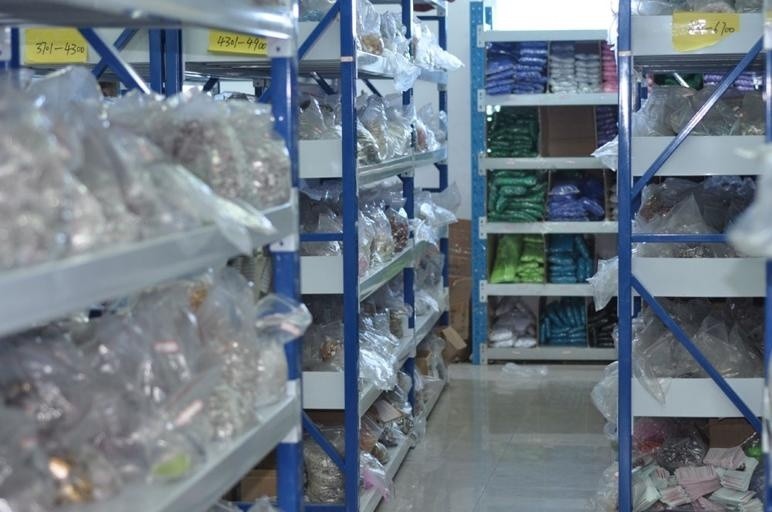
441,211 -> 476,360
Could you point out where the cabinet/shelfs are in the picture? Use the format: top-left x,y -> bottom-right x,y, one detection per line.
618,0 -> 772,511
471,1 -> 620,363
